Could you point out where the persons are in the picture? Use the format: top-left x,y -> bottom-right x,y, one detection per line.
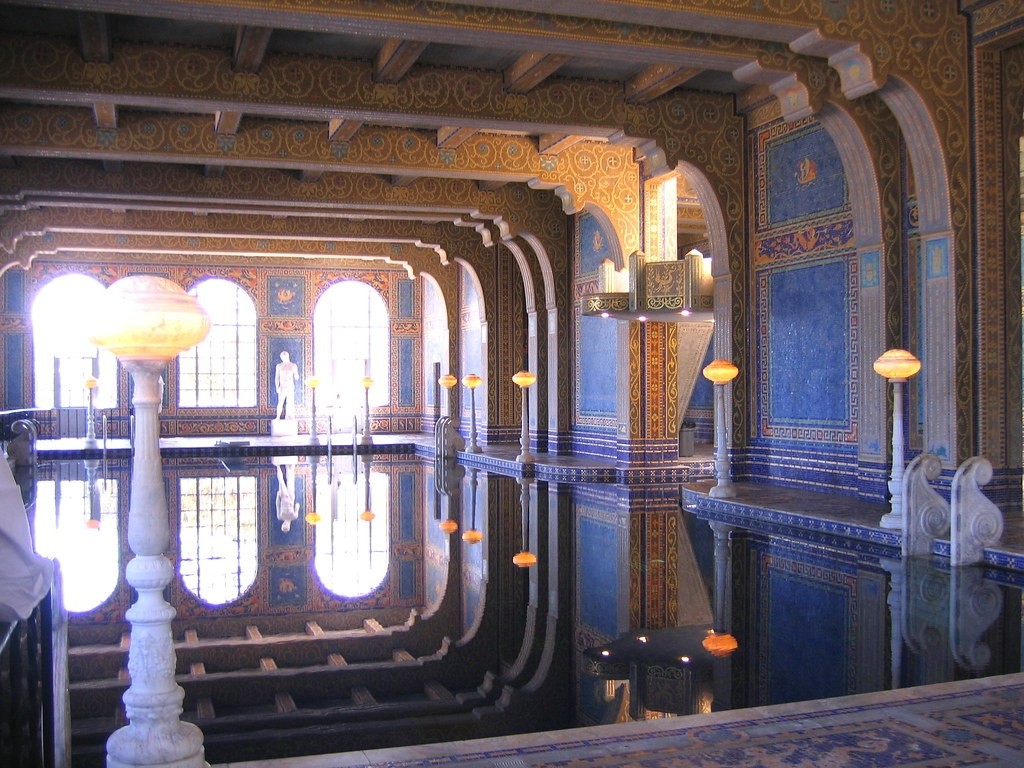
275,350 -> 300,419
275,465 -> 300,534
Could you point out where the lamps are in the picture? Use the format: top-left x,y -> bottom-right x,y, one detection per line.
873,348 -> 922,529
702,358 -> 739,496
305,456 -> 320,525
438,375 -> 457,416
79,274 -> 211,556
461,373 -> 483,453
512,477 -> 538,568
305,376 -> 321,445
462,466 -> 483,545
360,455 -> 375,521
512,371 -> 536,462
85,459 -> 100,530
702,522 -> 738,657
84,376 -> 99,449
439,495 -> 459,534
879,559 -> 902,689
361,378 -> 375,444
